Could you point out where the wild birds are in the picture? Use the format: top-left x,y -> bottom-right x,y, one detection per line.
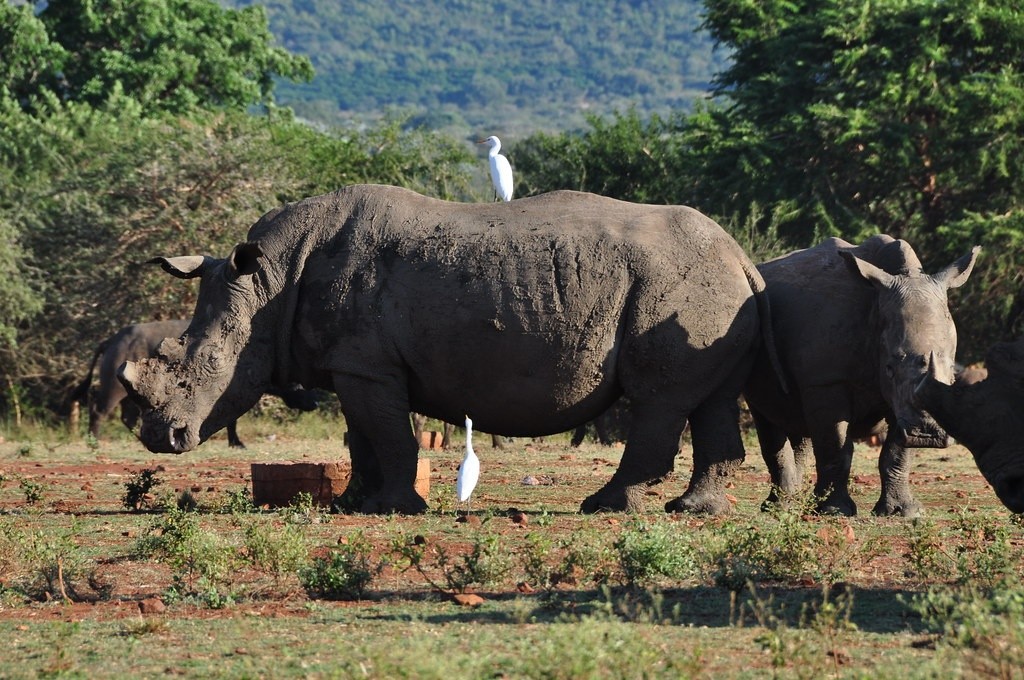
475,135 -> 514,203
452,415 -> 480,517
